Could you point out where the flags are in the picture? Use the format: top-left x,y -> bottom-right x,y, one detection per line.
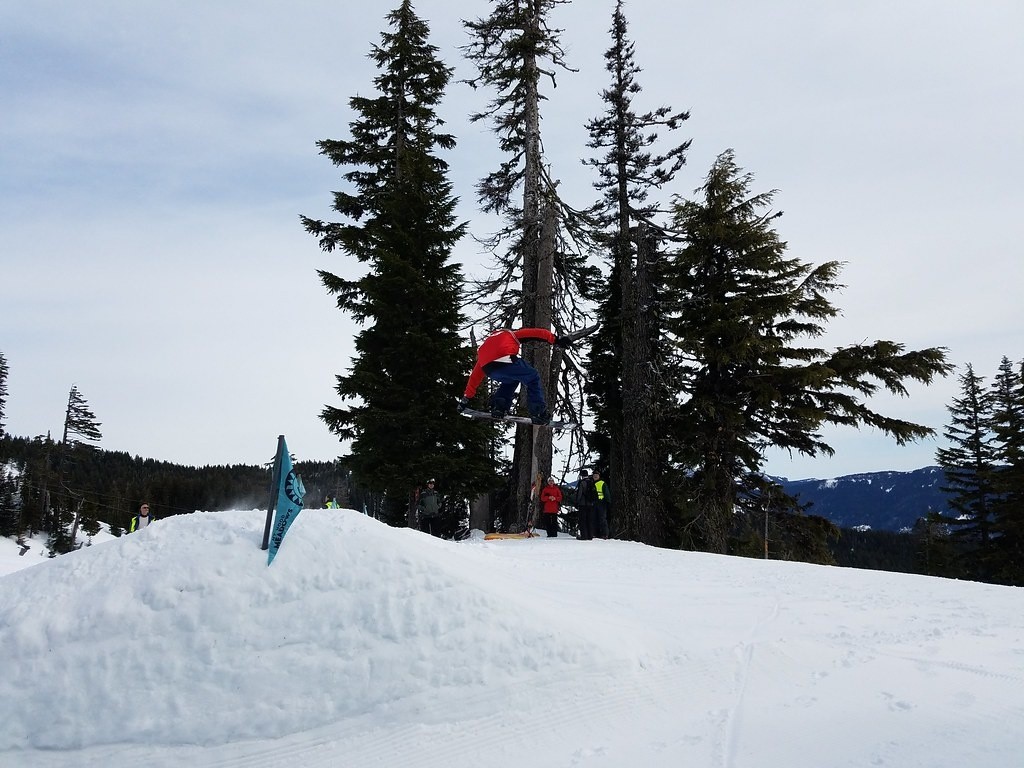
267,438 -> 305,566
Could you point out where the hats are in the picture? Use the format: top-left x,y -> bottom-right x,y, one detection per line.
592,470 -> 600,474
578,470 -> 588,476
547,477 -> 554,482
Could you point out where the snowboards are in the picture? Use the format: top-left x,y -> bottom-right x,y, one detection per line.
459,403 -> 585,432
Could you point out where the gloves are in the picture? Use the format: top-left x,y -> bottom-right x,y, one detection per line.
455,397 -> 468,413
555,335 -> 573,348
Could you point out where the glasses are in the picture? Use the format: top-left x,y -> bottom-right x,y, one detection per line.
141,506 -> 150,509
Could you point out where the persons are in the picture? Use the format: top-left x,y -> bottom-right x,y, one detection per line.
418,478 -> 442,537
542,477 -> 562,537
590,470 -> 610,539
129,504 -> 156,531
576,470 -> 597,540
457,327 -> 574,424
321,494 -> 341,511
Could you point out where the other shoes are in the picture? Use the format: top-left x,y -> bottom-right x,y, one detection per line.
492,403 -> 508,419
528,410 -> 551,423
577,533 -> 607,541
